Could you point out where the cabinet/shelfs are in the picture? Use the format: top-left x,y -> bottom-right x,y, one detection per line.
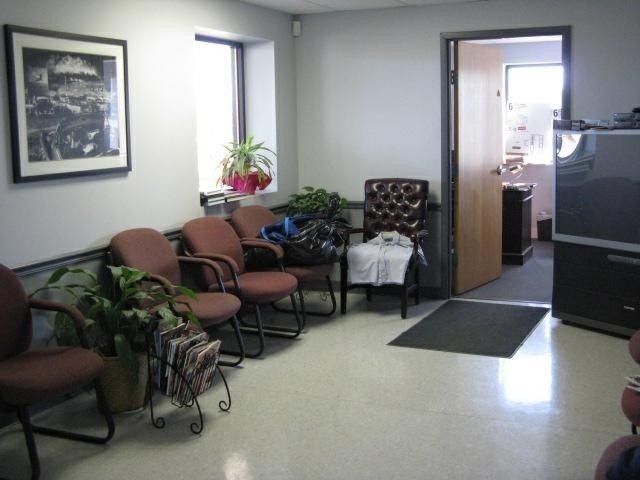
552,241 -> 640,337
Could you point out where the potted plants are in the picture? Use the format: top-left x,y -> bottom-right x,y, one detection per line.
27,265 -> 202,419
216,136 -> 279,195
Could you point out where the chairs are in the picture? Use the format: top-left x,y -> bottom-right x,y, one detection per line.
178,216 -> 302,358
627,330 -> 640,364
0,262 -> 115,480
621,376 -> 640,434
338,177 -> 429,320
595,434 -> 640,480
229,205 -> 336,334
106,228 -> 246,368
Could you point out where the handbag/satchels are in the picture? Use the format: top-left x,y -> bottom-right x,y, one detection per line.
247,213 -> 352,272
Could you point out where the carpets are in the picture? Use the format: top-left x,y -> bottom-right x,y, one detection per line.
386,299 -> 549,360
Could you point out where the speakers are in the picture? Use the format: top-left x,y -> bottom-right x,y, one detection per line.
292,20 -> 302,37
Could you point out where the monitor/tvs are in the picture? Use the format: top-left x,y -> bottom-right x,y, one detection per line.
551,128 -> 640,254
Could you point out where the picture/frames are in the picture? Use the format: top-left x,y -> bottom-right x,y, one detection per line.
4,24 -> 132,183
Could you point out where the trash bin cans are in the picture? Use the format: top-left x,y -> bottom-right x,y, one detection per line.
537,218 -> 552,241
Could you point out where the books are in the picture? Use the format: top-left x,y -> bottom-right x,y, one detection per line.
153,315 -> 221,404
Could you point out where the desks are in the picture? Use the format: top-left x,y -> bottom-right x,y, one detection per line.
502,186 -> 534,265
